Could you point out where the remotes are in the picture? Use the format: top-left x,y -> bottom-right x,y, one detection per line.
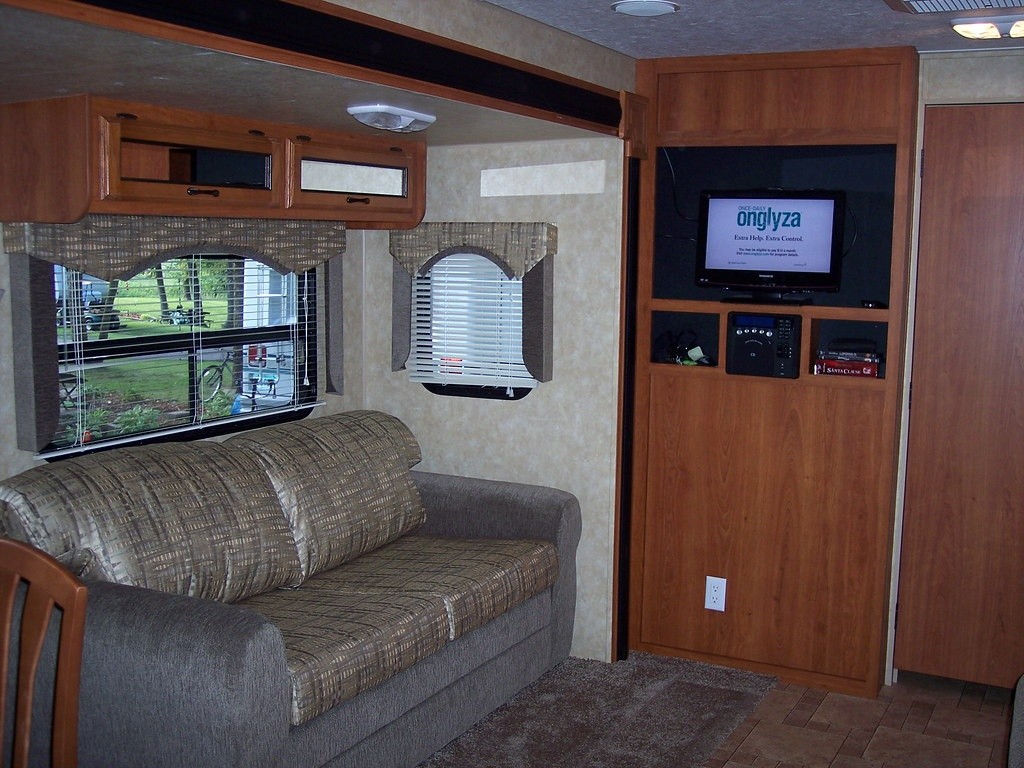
860,300 -> 890,309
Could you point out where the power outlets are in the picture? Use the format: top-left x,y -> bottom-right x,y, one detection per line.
705,576 -> 727,612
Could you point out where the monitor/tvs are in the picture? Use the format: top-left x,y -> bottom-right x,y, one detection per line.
695,189 -> 847,304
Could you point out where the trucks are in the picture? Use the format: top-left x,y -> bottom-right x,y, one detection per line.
239,258 -> 297,413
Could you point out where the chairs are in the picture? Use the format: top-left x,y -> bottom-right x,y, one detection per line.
0,539 -> 87,768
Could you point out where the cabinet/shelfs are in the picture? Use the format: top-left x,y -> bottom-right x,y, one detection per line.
0,95 -> 427,230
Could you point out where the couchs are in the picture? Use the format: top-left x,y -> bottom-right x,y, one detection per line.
0,409 -> 582,768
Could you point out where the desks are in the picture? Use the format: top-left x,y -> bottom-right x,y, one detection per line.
57,374 -> 89,411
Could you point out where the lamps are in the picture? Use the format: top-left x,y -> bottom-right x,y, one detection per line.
346,105 -> 437,133
950,14 -> 1024,40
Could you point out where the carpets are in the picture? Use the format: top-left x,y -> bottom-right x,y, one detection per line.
417,648 -> 776,768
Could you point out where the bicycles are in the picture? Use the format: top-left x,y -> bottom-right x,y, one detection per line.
197,347 -> 241,403
